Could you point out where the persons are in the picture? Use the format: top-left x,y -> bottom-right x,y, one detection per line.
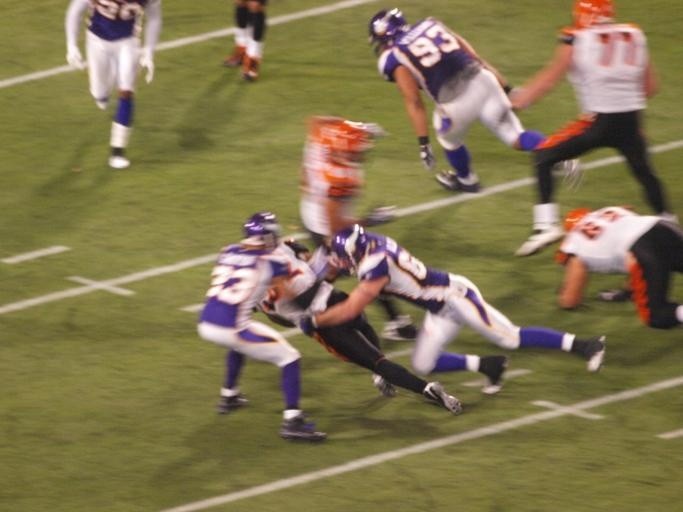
298,224 -> 606,397
367,8 -> 585,189
196,208 -> 326,441
554,205 -> 682,329
63,0 -> 159,171
297,113 -> 394,239
508,2 -> 668,259
225,1 -> 268,80
257,238 -> 462,414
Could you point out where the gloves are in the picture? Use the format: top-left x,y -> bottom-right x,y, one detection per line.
417,135 -> 435,168
139,56 -> 153,85
66,44 -> 83,69
298,315 -> 319,338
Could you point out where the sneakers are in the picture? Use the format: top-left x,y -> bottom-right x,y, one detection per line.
515,224 -> 563,257
223,52 -> 261,80
219,394 -> 249,415
422,381 -> 463,416
107,153 -> 130,172
380,323 -> 417,341
585,334 -> 605,373
373,374 -> 396,396
434,171 -> 481,192
478,355 -> 509,394
277,415 -> 327,440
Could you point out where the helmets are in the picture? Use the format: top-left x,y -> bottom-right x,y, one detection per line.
243,213 -> 367,268
565,208 -> 590,231
370,6 -> 409,40
573,0 -> 614,28
